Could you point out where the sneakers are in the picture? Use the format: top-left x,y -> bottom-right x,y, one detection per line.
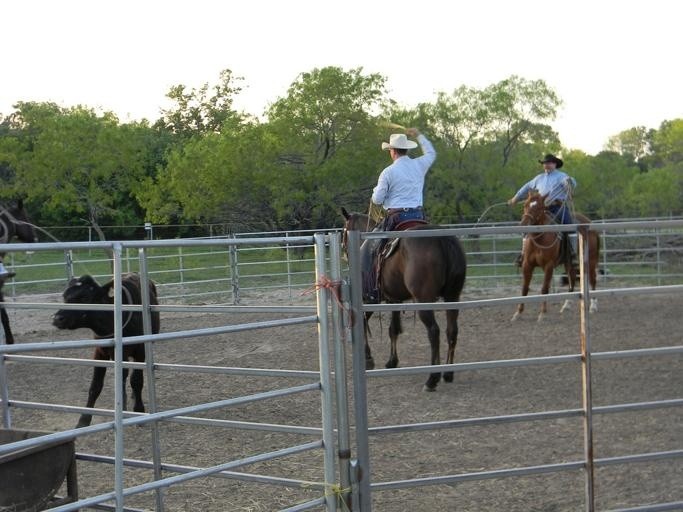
571,254 -> 579,266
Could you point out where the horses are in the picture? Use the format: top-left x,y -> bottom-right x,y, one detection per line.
509,186 -> 601,323
340,204 -> 467,393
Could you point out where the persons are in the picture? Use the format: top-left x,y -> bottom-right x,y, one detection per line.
508,152 -> 577,232
359,125 -> 437,290
0,252 -> 15,345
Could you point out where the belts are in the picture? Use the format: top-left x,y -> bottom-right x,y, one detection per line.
387,205 -> 424,215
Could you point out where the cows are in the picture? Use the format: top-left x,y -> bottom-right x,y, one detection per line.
51,271 -> 160,431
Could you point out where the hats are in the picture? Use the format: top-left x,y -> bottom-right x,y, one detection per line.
381,133 -> 419,151
538,153 -> 564,169
